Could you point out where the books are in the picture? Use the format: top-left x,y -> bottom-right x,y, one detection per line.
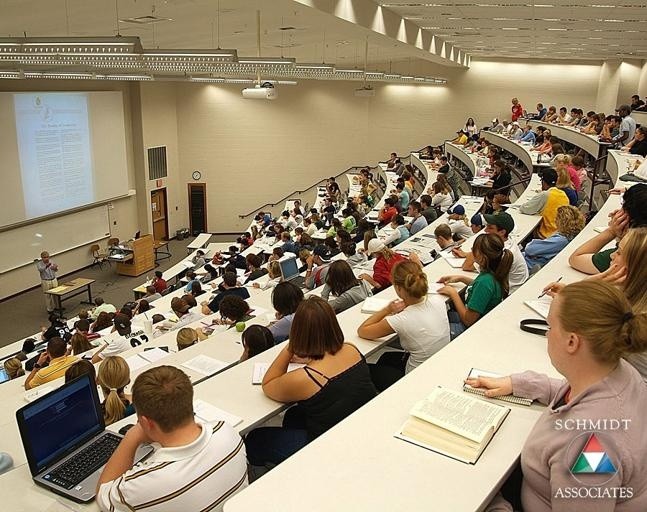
390,385 -> 511,465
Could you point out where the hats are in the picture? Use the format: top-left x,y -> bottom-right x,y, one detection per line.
171,297 -> 191,313
615,104 -> 632,112
368,238 -> 385,256
453,205 -> 464,215
115,315 -> 131,336
492,118 -> 498,123
456,128 -> 463,133
471,212 -> 514,232
314,245 -> 332,263
538,169 -> 558,185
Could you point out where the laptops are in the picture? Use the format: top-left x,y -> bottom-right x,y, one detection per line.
16,371 -> 154,503
0,366 -> 9,384
279,255 -> 306,289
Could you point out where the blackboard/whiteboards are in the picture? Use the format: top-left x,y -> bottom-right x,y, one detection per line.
0,203 -> 111,275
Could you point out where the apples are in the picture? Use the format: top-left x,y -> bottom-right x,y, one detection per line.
235,321 -> 245,332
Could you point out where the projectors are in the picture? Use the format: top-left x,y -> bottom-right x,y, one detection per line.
242,84 -> 278,100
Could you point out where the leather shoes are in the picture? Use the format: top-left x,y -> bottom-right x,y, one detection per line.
48,308 -> 66,314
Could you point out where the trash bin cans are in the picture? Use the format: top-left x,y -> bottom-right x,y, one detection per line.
177,228 -> 190,240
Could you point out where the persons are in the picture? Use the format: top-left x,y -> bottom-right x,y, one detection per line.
463,276 -> 646,512
93,354 -> 137,427
2,94 -> 646,389
244,293 -> 378,468
94,364 -> 249,511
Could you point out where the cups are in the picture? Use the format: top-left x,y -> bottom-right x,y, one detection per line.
536,153 -> 541,164
144,318 -> 154,333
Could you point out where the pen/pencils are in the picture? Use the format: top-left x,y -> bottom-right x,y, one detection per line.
537,277 -> 562,298
448,247 -> 461,253
